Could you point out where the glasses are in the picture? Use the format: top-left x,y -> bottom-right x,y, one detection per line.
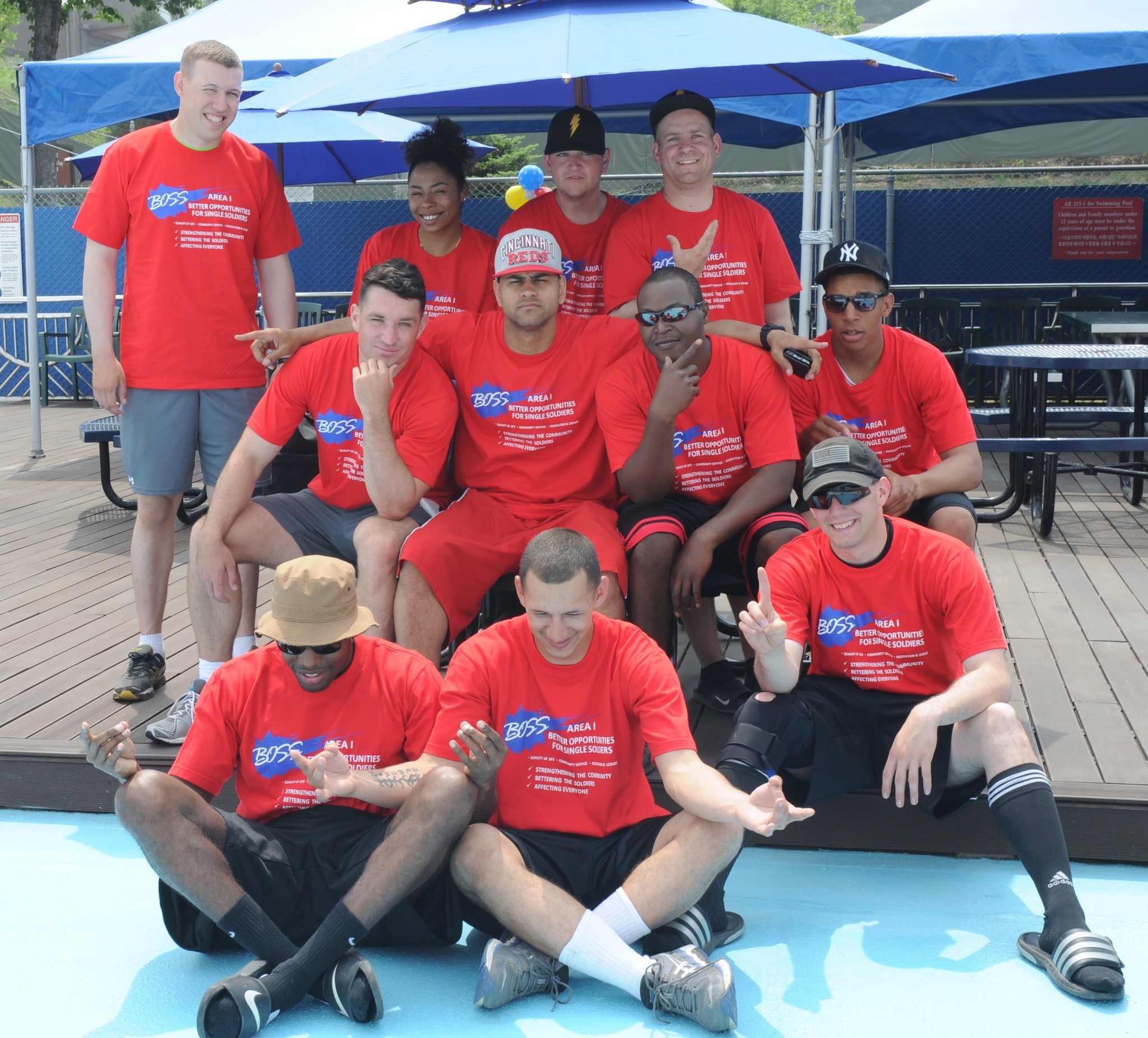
276,640 -> 341,655
806,483 -> 871,510
635,301 -> 703,327
822,290 -> 888,314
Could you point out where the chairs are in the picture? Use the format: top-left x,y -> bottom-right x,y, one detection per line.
900,297 -> 969,403
974,297 -> 1042,409
1055,295 -> 1148,406
38,306 -> 121,409
262,302 -> 350,392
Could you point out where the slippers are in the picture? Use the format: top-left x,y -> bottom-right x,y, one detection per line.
652,905 -> 747,957
196,958 -> 274,1038
1016,928 -> 1126,1000
313,946 -> 385,1024
692,679 -> 755,714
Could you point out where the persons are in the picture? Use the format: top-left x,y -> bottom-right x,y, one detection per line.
77,44 -> 1130,1037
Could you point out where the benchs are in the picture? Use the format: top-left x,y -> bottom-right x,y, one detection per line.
78,414 -> 140,510
966,407 -> 1148,536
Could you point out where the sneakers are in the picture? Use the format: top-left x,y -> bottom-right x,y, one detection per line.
643,945 -> 739,1032
145,678 -> 208,745
112,645 -> 168,700
473,927 -> 573,1014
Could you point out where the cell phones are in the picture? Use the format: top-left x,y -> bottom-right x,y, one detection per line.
784,349 -> 811,380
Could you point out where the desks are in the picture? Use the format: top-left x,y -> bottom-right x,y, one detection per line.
1059,310 -> 1148,433
964,344 -> 1148,505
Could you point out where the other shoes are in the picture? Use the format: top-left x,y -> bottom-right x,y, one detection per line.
642,742 -> 656,775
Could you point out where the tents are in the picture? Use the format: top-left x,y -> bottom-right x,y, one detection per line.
822,1 -> 1147,334
15,0 -> 815,470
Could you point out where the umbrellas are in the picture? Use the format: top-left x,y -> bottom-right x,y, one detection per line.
61,0 -> 957,351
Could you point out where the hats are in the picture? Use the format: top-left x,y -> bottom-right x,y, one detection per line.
492,228 -> 564,280
815,239 -> 893,291
254,555 -> 380,647
544,105 -> 607,155
649,89 -> 716,130
801,436 -> 886,501
